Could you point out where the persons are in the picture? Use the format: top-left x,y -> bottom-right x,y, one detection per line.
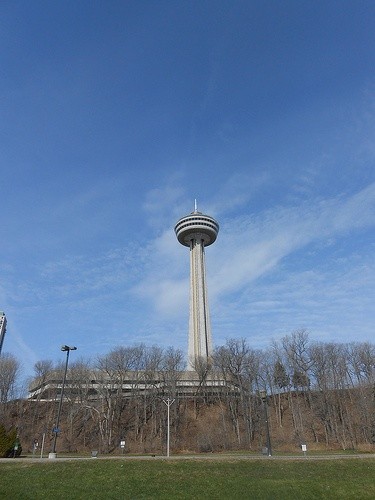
33,439 -> 39,458
13,438 -> 20,459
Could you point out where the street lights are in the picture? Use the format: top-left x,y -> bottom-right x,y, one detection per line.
260,389 -> 272,457
48,344 -> 77,458
